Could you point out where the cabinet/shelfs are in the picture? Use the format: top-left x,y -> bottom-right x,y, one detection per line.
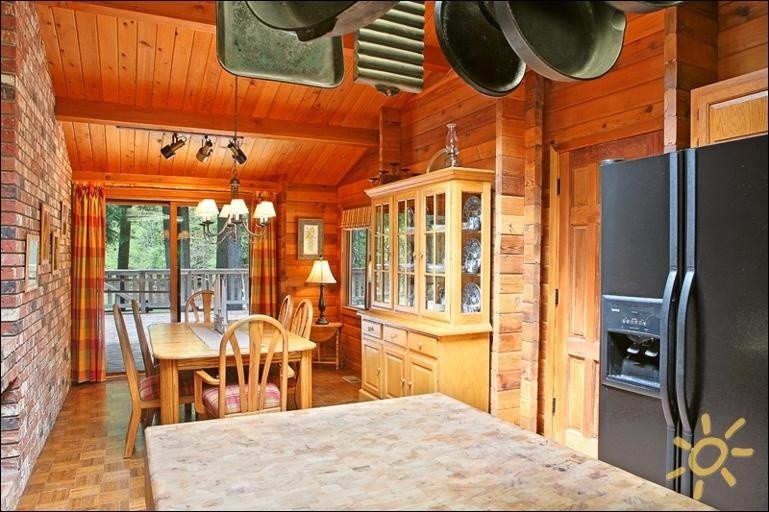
395,166 -> 495,323
383,319 -> 492,413
366,180 -> 394,312
357,311 -> 383,402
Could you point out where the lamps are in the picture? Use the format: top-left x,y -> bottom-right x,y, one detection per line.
305,257 -> 338,325
194,75 -> 280,249
155,131 -> 248,170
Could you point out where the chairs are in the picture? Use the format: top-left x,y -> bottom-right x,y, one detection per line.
112,289 -> 318,460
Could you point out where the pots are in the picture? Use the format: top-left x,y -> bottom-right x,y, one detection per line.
432,0 -> 629,99
245,1 -> 396,43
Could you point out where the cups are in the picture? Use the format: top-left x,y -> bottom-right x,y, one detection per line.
433,303 -> 445,311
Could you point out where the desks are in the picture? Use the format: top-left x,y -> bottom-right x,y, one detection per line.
308,323 -> 343,369
143,388 -> 725,512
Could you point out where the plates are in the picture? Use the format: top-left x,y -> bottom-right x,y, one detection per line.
463,282 -> 480,310
462,238 -> 480,274
464,197 -> 481,231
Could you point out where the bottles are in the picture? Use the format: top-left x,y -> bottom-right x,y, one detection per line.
213,309 -> 227,334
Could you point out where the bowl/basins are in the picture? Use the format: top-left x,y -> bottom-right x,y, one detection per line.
429,224 -> 444,230
428,261 -> 443,270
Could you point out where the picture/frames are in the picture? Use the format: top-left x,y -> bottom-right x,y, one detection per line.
296,216 -> 324,260
21,199 -> 70,297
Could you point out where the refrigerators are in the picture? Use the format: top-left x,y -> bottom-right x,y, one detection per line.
597,136 -> 767,510
